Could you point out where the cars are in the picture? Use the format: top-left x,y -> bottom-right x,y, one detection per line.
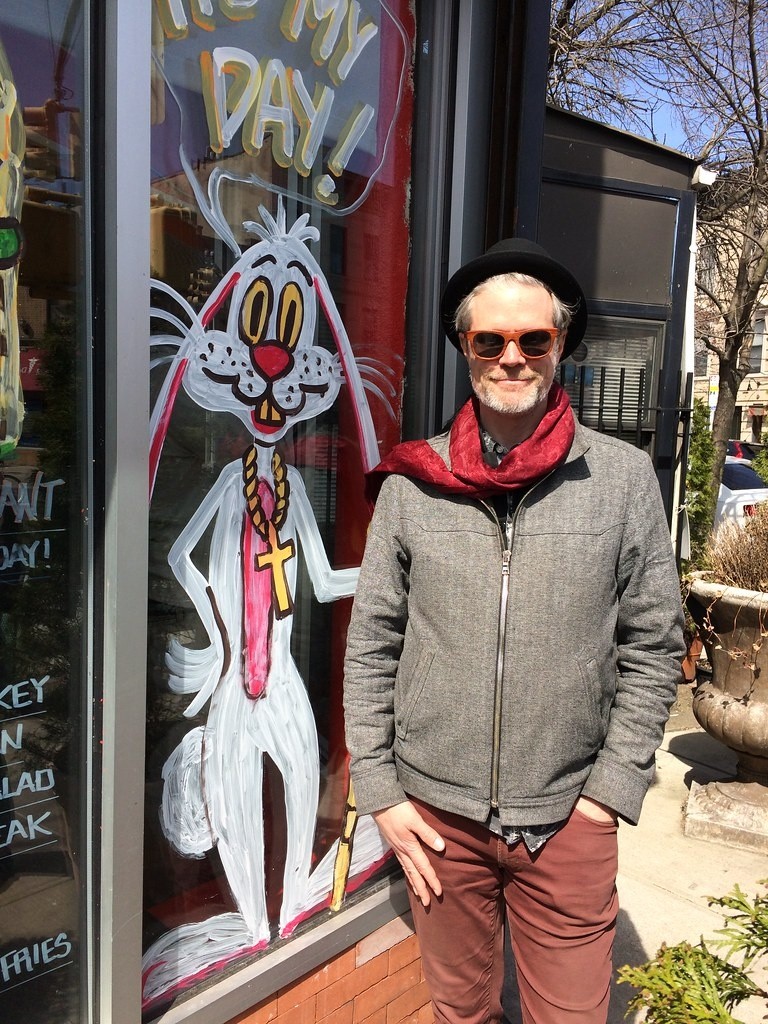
685,455 -> 768,562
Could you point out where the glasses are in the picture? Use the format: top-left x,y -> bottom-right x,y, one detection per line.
465,328 -> 562,360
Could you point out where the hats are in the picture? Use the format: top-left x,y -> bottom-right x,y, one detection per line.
439,238 -> 588,363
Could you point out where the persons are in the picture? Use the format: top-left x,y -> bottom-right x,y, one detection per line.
342,241 -> 686,1024
